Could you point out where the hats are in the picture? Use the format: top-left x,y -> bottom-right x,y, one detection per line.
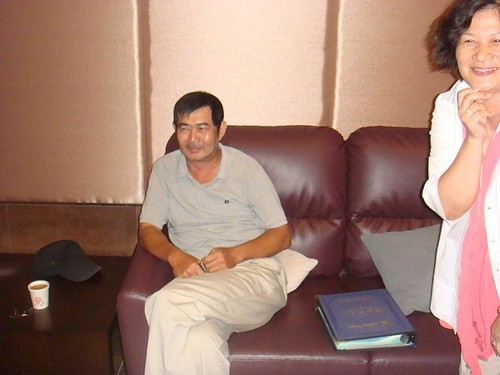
33,239 -> 103,282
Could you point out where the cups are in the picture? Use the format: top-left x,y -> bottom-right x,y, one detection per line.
28,280 -> 49,309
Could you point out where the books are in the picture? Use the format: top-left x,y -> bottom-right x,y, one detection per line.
315,288 -> 416,351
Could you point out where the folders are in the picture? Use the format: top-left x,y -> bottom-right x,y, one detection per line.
316,289 -> 417,351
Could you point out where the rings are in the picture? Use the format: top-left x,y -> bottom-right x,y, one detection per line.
491,342 -> 495,346
465,108 -> 473,116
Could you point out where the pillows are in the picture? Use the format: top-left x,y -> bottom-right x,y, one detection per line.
361,222 -> 441,318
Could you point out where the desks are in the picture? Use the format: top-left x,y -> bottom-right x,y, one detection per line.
0,251 -> 134,375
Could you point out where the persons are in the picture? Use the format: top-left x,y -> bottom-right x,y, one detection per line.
421,0 -> 500,375
139,90 -> 292,375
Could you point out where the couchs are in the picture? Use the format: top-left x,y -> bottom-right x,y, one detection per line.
116,124 -> 463,374
